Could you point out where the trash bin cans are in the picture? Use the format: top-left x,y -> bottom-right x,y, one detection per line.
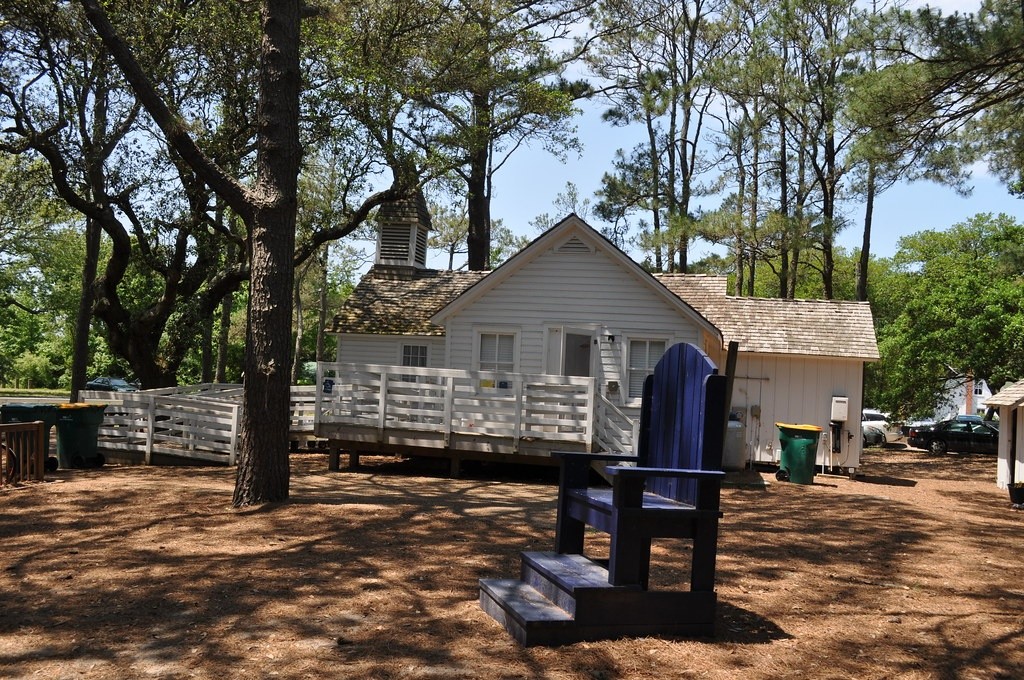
0,402 -> 58,482
55,403 -> 107,469
778,422 -> 824,486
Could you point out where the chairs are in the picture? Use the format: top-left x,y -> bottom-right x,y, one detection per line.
550,343 -> 728,587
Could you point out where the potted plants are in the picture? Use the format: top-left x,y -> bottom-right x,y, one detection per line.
1007,482 -> 1024,505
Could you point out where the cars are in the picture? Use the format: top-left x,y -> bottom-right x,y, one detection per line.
84,377 -> 139,394
953,413 -> 983,429
129,377 -> 143,391
863,408 -> 903,444
907,419 -> 999,455
860,424 -> 886,448
